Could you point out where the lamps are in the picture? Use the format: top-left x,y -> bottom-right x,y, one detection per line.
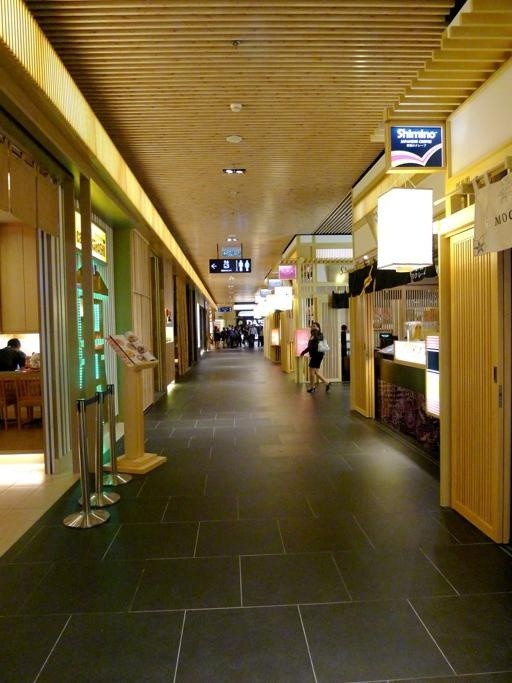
375,173 -> 434,272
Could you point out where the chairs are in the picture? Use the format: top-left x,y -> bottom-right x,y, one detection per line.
0,370 -> 43,432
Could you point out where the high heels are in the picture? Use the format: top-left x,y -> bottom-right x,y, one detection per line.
308,387 -> 315,392
325,383 -> 333,391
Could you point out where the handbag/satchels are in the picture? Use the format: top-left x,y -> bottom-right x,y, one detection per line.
318,339 -> 330,352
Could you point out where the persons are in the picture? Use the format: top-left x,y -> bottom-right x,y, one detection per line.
214,320 -> 263,350
0,337 -> 26,371
299,321 -> 332,393
